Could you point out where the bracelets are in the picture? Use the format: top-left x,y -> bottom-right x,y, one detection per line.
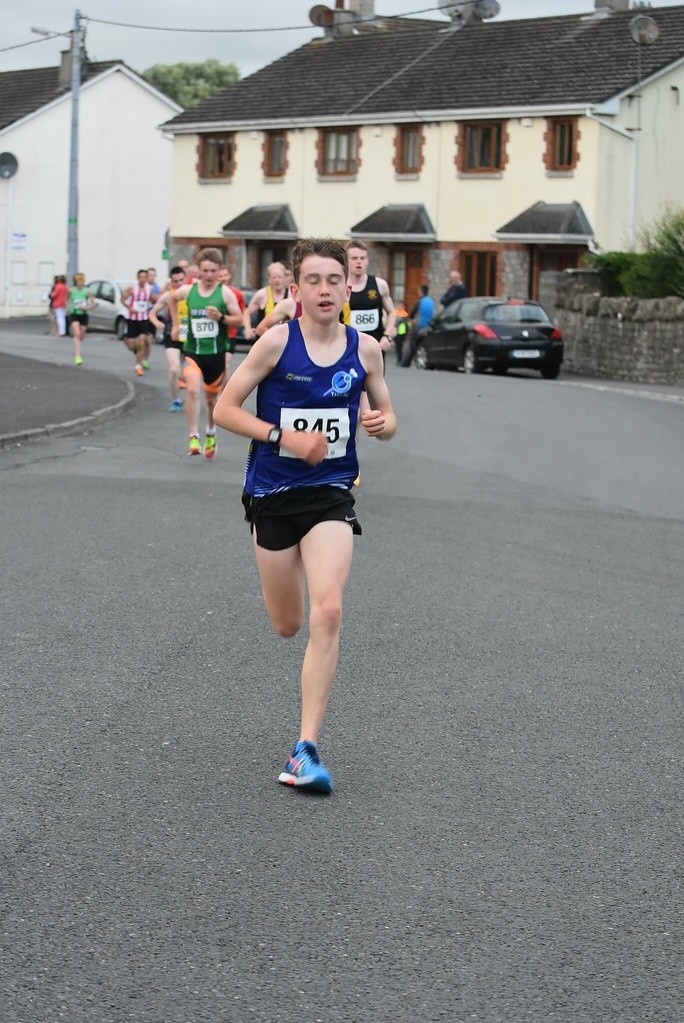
218,314 -> 224,322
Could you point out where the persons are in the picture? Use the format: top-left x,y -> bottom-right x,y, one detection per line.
399,284 -> 434,367
49,247 -> 302,459
339,240 -> 396,426
440,270 -> 465,307
213,239 -> 398,795
394,300 -> 409,362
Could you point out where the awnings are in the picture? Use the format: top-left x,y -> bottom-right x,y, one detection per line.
347,204 -> 436,242
494,200 -> 593,243
222,204 -> 298,240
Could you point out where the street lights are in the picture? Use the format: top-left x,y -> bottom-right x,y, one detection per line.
28,25 -> 84,288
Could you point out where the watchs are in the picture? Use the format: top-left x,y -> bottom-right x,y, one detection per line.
382,334 -> 392,342
267,426 -> 282,448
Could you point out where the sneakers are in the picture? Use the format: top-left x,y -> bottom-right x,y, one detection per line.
278,741 -> 333,793
204,425 -> 217,458
75,355 -> 82,365
170,397 -> 185,412
178,377 -> 186,389
188,434 -> 202,456
135,366 -> 144,376
142,361 -> 148,369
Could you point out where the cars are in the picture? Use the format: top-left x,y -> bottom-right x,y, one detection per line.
150,283 -> 262,353
83,278 -> 135,339
413,296 -> 565,379
